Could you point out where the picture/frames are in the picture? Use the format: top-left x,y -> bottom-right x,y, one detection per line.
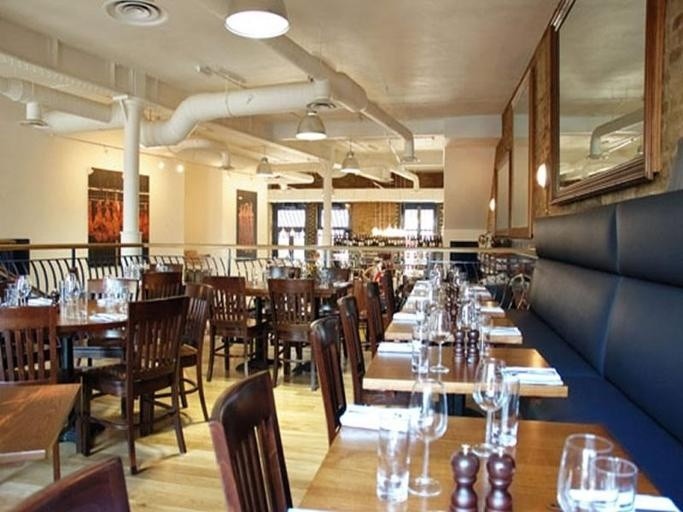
236,189 -> 258,259
88,168 -> 150,266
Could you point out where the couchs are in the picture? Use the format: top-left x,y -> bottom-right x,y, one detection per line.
504,203 -> 617,387
523,190 -> 683,511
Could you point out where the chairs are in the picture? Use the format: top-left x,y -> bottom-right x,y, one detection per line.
366,281 -> 385,359
150,281 -> 213,432
203,276 -> 268,382
142,272 -> 181,300
321,267 -> 350,358
383,270 -> 397,322
269,266 -> 300,279
0,306 -> 57,386
337,295 -> 421,407
87,278 -> 138,366
82,294 -> 189,475
207,369 -> 294,512
266,278 -> 319,390
309,316 -> 346,446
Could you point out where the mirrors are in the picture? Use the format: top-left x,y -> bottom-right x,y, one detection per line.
548,0 -> 665,206
509,66 -> 533,240
493,149 -> 510,237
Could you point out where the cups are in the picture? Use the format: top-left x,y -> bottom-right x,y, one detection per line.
497,377 -> 518,447
555,434 -> 612,512
374,411 -> 410,502
471,359 -> 506,457
16,275 -> 28,308
430,312 -> 451,373
413,326 -> 427,374
7,289 -> 18,307
407,381 -> 447,497
587,457 -> 638,512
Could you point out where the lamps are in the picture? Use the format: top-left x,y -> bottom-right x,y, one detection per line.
224,0 -> 292,39
536,160 -> 551,188
256,145 -> 272,176
295,108 -> 327,140
340,145 -> 360,173
